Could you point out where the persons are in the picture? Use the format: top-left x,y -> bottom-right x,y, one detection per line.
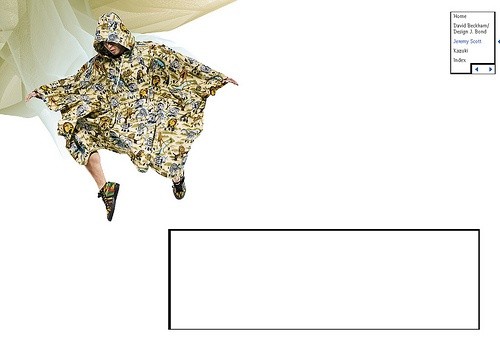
26,11 -> 238,221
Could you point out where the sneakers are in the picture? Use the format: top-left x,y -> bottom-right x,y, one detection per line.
172,177 -> 186,200
98,181 -> 119,221
